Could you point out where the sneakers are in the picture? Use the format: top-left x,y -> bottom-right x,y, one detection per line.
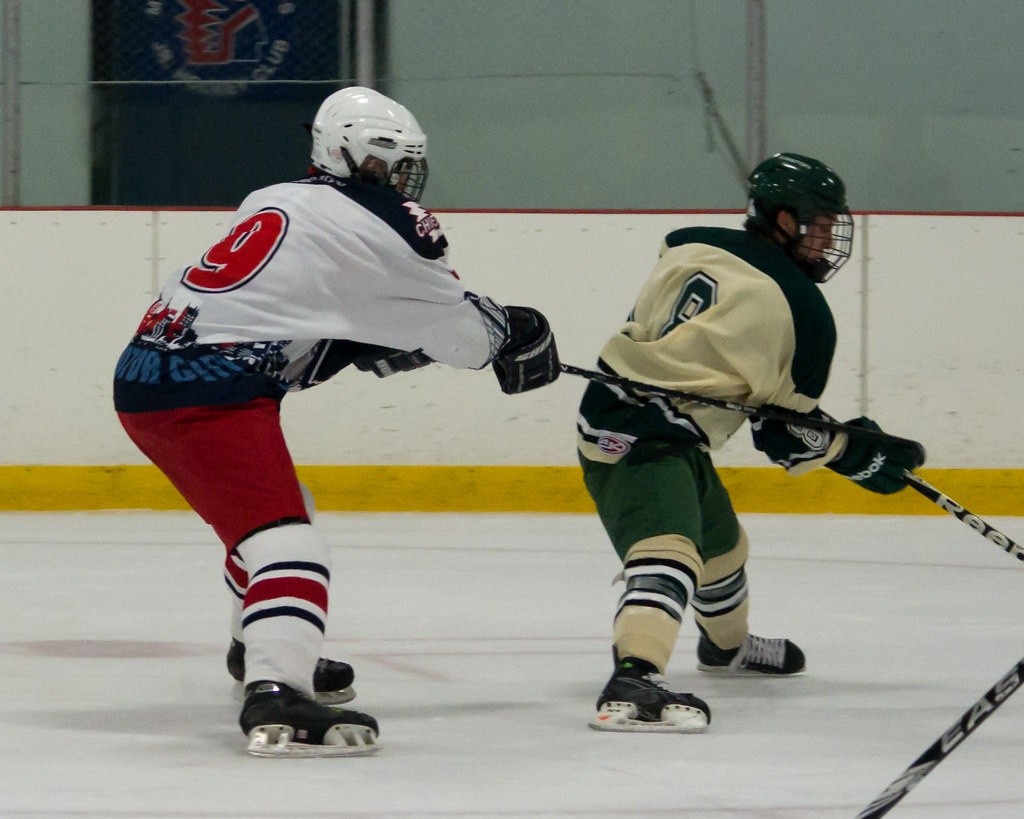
697,633 -> 806,675
240,680 -> 378,759
227,639 -> 357,705
587,663 -> 712,732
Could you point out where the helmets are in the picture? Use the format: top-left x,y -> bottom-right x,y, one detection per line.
309,85 -> 429,204
747,151 -> 855,285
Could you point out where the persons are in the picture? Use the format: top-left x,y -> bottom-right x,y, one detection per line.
571,150 -> 929,730
106,85 -> 559,753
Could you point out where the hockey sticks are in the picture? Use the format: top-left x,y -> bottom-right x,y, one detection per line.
905,472 -> 1023,559
852,657 -> 1024,819
558,360 -> 928,468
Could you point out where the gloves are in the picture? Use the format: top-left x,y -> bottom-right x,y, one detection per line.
371,346 -> 434,378
492,306 -> 560,394
823,416 -> 926,495
